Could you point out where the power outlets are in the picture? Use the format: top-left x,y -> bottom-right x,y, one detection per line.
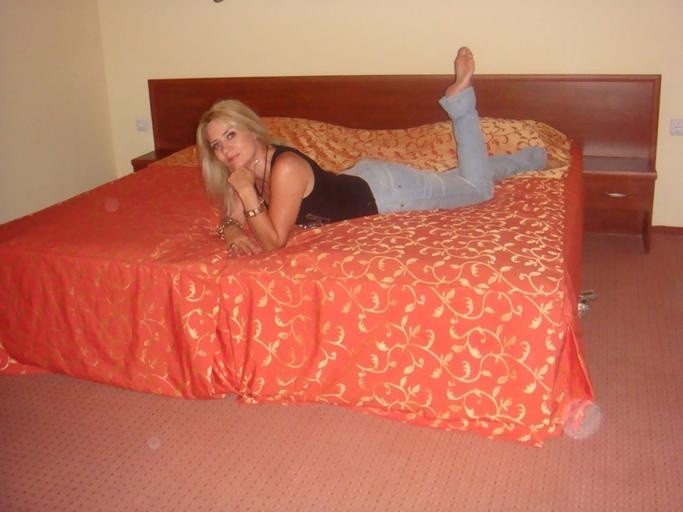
670,118 -> 683,136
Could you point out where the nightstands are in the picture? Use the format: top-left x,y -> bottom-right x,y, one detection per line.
131,150 -> 174,172
581,157 -> 658,254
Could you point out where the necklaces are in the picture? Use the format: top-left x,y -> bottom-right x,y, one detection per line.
254,144 -> 268,203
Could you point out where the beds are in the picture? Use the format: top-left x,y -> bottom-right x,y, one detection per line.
0,75 -> 661,448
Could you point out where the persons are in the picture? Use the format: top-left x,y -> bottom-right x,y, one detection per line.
192,45 -> 571,269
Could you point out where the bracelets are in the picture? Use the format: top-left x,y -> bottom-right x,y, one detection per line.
213,217 -> 246,239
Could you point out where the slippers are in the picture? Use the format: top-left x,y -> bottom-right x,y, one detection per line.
577,289 -> 598,319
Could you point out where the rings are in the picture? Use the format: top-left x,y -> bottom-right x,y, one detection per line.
229,243 -> 236,249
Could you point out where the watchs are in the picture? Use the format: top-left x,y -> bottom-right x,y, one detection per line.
243,205 -> 266,219
